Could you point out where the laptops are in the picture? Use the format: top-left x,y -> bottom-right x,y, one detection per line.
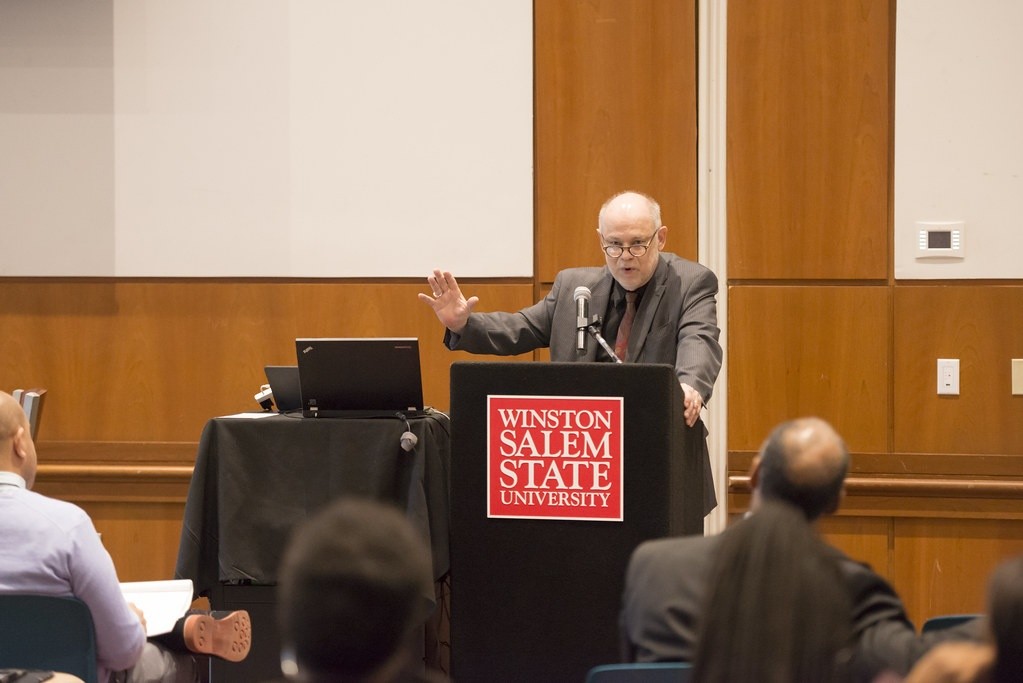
295,337 -> 433,417
264,366 -> 303,413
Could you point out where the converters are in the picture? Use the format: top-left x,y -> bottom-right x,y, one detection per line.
255,388 -> 276,410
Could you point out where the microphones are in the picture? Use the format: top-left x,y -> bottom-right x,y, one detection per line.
574,286 -> 591,355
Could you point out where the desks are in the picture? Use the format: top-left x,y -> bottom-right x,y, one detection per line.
171,411 -> 447,683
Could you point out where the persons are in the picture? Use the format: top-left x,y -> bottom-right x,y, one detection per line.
418,193 -> 724,426
623,416 -> 917,683
0,392 -> 251,683
275,499 -> 432,683
866,561 -> 1023,682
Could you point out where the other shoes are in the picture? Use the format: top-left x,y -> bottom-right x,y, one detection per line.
165,610 -> 252,664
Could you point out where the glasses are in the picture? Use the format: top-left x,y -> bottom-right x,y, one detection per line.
602,227 -> 659,259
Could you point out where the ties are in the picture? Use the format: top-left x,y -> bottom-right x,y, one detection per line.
614,292 -> 637,361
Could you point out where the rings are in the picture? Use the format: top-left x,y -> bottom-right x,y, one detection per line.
433,292 -> 443,298
